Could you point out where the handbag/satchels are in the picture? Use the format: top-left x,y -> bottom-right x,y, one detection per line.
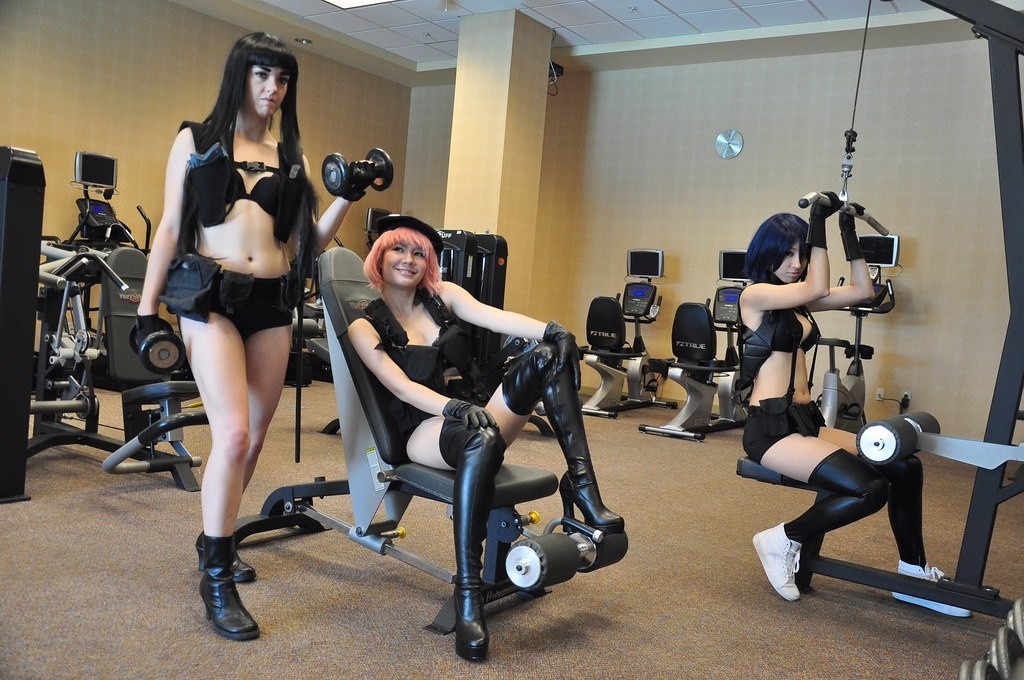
158,255 -> 222,322
790,400 -> 825,437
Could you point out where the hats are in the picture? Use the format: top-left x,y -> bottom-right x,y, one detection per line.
377,214 -> 443,253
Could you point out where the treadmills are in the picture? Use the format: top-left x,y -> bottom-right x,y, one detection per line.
60,148 -> 195,394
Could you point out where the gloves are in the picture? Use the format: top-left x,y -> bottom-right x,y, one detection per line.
342,160 -> 377,202
805,191 -> 845,249
543,320 -> 581,389
839,202 -> 865,261
134,314 -> 165,346
443,399 -> 500,431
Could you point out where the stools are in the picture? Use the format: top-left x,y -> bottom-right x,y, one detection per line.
736,455 -> 839,591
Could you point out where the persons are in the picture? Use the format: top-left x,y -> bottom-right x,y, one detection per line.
135,32 -> 376,646
736,189 -> 973,620
345,215 -> 626,661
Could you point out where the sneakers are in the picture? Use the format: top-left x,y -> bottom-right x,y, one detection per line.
892,562 -> 970,617
753,523 -> 801,601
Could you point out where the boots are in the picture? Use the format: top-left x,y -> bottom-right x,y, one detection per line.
439,422 -> 506,662
196,534 -> 256,582
200,532 -> 260,641
502,343 -> 624,533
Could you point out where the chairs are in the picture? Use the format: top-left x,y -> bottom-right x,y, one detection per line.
318,248 -> 559,636
659,302 -> 738,432
103,246 -> 210,492
582,297 -> 642,411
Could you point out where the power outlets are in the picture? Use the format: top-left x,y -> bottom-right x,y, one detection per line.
901,390 -> 912,400
875,388 -> 884,401
